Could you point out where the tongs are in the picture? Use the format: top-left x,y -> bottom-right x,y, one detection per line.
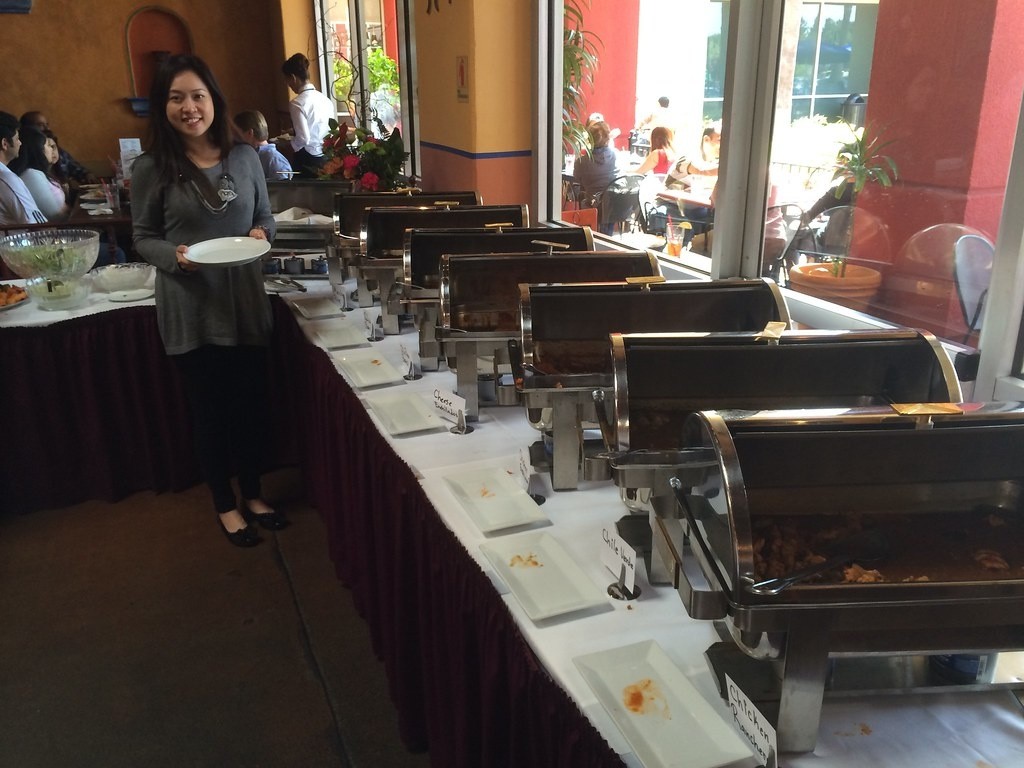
749,550 -> 857,595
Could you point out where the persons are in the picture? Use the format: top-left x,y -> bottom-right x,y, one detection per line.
338,84 -> 402,141
631,96 -> 721,253
128,54 -> 286,547
572,113 -> 622,236
0,111 -> 126,273
783,144 -> 860,291
273,53 -> 334,178
689,166 -> 787,276
233,109 -> 293,181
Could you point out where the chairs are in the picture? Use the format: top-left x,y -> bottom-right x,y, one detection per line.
952,233 -> 995,344
593,172 -> 853,288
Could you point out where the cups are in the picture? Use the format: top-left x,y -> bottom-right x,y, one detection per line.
105,185 -> 120,217
564,154 -> 575,175
666,222 -> 685,259
311,259 -> 329,275
284,258 -> 304,274
264,258 -> 280,274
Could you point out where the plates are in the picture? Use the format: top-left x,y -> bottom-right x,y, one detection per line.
573,639 -> 754,768
441,464 -> 548,532
315,325 -> 366,349
0,298 -> 31,310
292,297 -> 344,319
337,353 -> 404,388
86,189 -> 97,192
79,184 -> 106,188
80,195 -> 106,201
479,531 -> 610,621
183,237 -> 271,267
365,392 -> 445,435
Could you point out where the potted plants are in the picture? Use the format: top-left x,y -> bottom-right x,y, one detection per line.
788,115 -> 899,331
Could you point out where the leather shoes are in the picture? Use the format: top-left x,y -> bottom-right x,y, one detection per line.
240,498 -> 288,530
217,513 -> 260,547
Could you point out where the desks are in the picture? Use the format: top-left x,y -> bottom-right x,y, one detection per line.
0,270 -> 1024,768
657,187 -> 715,257
561,168 -> 582,211
68,181 -> 132,265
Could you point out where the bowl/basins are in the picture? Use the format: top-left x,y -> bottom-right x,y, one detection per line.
0,230 -> 100,281
90,263 -> 157,301
25,272 -> 93,310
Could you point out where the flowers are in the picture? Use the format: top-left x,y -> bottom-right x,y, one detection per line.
298,117 -> 412,194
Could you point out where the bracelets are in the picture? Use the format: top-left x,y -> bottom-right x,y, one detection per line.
251,225 -> 271,241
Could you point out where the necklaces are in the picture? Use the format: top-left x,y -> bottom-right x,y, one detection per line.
179,155 -> 238,219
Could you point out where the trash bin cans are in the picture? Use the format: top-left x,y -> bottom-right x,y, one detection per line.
841,93 -> 866,131
